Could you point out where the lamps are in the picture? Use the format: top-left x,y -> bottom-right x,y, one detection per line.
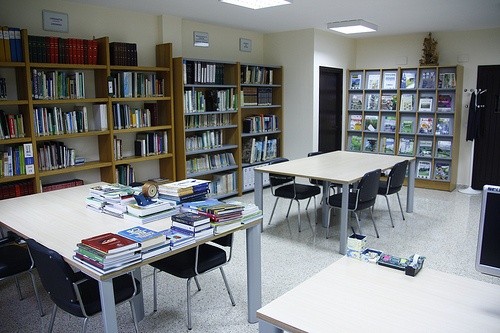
328,20 -> 377,34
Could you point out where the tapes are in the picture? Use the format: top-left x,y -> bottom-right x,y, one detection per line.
409,254 -> 421,266
142,182 -> 158,197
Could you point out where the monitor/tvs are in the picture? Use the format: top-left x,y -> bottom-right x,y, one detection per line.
475,184 -> 500,277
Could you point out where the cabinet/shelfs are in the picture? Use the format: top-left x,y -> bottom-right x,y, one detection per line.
0,29 -> 284,200
345,65 -> 464,192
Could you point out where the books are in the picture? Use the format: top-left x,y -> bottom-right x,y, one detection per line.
382,95 -> 396,110
419,98 -> 432,112
364,115 -> 377,129
382,116 -> 395,130
367,94 -> 379,110
365,138 -> 377,150
438,72 -> 455,88
384,73 -> 396,89
350,115 -> 362,129
0,26 -> 278,200
367,75 -> 379,89
383,118 -> 451,180
401,72 -> 415,88
351,137 -> 361,150
438,95 -> 451,112
422,72 -> 435,88
350,74 -> 362,89
350,95 -> 362,110
400,94 -> 412,109
73,178 -> 263,275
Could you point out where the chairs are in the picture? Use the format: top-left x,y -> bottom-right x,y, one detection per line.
0,227 -> 235,333
270,151 -> 408,243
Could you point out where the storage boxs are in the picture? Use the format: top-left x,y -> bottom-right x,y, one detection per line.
348,234 -> 367,252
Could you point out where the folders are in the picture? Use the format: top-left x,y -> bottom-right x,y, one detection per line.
8,27 -> 17,62
15,28 -> 23,62
2,26 -> 12,62
0,26 -> 6,62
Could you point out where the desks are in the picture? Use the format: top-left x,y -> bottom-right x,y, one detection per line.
0,182 -> 263,333
253,150 -> 415,256
256,254 -> 500,333
